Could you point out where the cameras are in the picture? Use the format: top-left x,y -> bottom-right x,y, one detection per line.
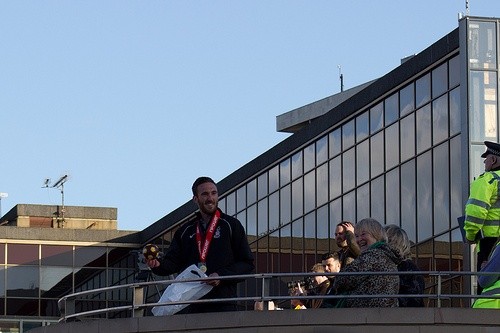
287,276 -> 314,290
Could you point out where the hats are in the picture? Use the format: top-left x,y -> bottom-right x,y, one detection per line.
481,141 -> 500,158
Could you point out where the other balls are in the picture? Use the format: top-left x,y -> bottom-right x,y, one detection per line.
143,243 -> 159,260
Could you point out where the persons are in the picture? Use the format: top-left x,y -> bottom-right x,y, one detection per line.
334,219 -> 400,309
472,239 -> 500,309
290,219 -> 425,309
144,177 -> 255,315
463,154 -> 500,295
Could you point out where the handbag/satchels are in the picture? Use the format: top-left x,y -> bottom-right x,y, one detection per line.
151,264 -> 214,316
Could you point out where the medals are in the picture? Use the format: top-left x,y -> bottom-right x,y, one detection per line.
200,265 -> 207,273
